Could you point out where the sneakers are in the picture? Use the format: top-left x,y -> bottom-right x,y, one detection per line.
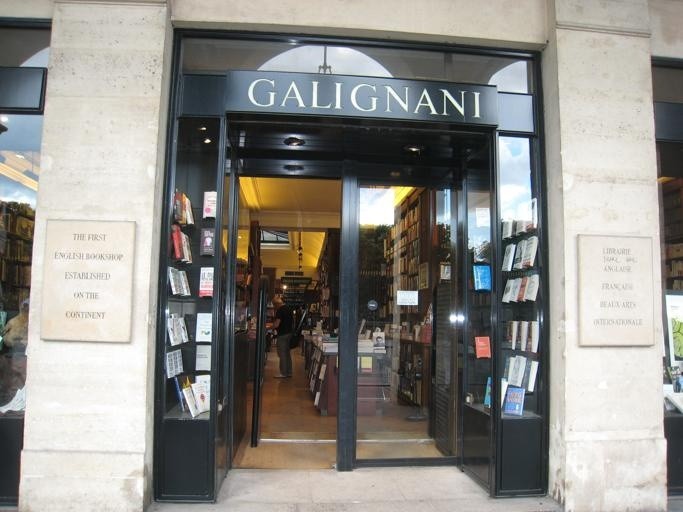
274,374 -> 291,378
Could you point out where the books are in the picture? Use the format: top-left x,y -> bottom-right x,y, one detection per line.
267,299 -> 277,344
0,201 -> 30,319
166,191 -> 215,417
310,288 -> 330,316
384,208 -> 429,405
301,316 -> 384,405
662,188 -> 682,295
473,199 -> 537,415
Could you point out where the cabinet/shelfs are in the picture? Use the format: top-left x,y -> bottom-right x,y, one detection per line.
0,203 -> 34,317
153,180 -> 552,507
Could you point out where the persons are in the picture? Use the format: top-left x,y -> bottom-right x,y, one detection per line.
267,297 -> 293,378
